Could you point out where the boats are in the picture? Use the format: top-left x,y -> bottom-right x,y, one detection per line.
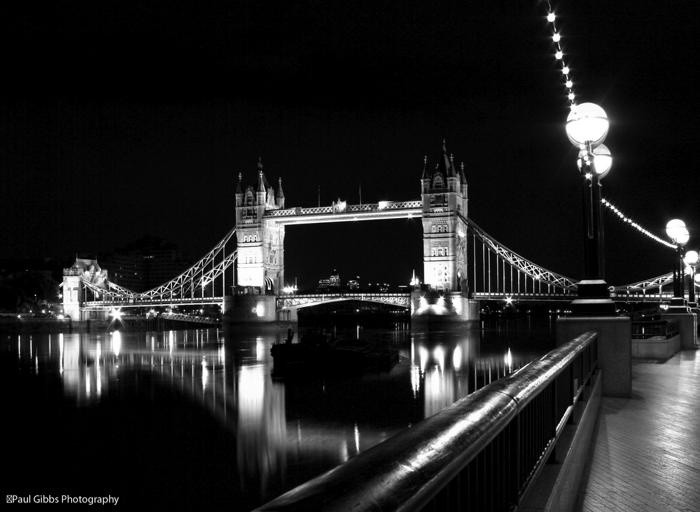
267,300 -> 412,364
123,304 -> 161,328
16,303 -> 71,329
161,304 -> 223,325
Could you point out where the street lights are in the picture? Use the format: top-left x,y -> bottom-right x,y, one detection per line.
665,216 -> 698,307
572,143 -> 616,281
563,101 -> 617,315
